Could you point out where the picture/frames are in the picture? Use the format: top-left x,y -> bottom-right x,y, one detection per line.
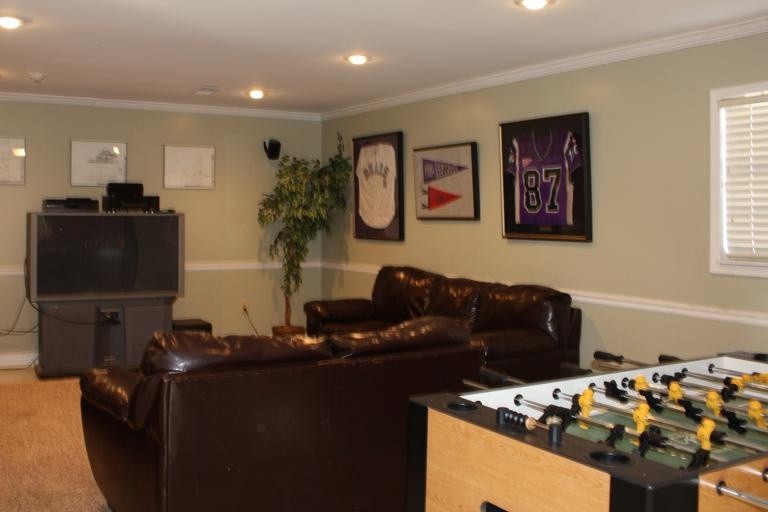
70,138 -> 127,188
0,136 -> 26,187
351,131 -> 404,242
412,140 -> 480,221
498,112 -> 592,242
163,143 -> 217,191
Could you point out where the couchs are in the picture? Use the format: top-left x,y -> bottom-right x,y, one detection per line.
305,265 -> 584,369
80,320 -> 563,510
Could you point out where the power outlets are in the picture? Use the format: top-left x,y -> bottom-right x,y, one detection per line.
240,299 -> 249,314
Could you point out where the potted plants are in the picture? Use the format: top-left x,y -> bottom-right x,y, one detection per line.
255,130 -> 352,343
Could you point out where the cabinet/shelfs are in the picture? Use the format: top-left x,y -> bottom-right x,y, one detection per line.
34,296 -> 176,381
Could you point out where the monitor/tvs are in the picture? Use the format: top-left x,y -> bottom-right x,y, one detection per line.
27,211 -> 185,301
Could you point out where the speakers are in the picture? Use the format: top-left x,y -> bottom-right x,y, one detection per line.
264,140 -> 281,161
